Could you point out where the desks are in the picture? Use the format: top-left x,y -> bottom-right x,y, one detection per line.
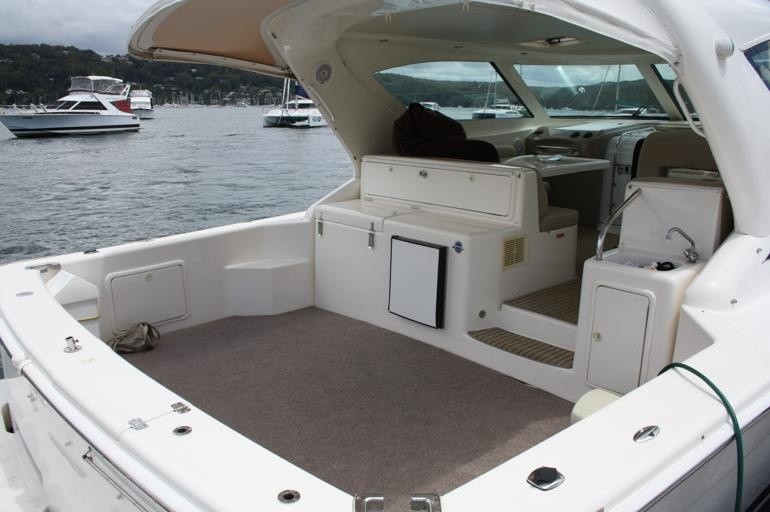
502,155 -> 614,223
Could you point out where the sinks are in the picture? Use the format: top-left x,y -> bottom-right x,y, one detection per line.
606,252 -> 684,272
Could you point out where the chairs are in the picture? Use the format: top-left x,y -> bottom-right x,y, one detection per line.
638,132 -> 720,177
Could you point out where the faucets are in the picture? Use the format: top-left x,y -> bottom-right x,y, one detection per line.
665,227 -> 694,251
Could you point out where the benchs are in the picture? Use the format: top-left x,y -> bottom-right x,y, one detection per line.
314,154 -> 580,303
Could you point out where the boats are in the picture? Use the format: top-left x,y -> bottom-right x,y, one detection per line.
0,75 -> 145,138
0,0 -> 769,512
128,88 -> 154,119
261,92 -> 331,129
